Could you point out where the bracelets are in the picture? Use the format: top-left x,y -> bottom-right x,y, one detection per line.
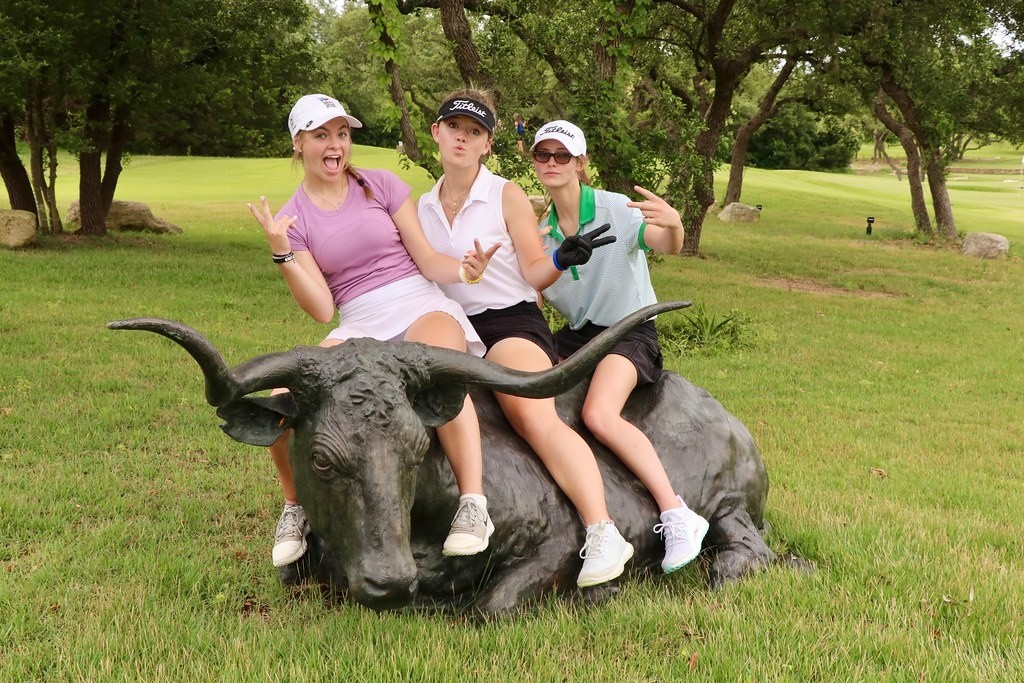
271,250 -> 296,265
458,261 -> 486,287
552,249 -> 570,272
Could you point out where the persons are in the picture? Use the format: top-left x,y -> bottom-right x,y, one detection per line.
415,91 -> 634,588
247,93 -> 502,568
514,115 -> 527,153
532,120 -> 709,575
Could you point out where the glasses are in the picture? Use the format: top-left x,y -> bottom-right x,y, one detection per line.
533,150 -> 573,164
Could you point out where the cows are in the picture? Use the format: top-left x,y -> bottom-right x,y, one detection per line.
104,301 -> 821,625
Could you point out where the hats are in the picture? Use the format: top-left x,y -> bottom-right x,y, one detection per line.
437,97 -> 495,132
529,120 -> 586,157
288,94 -> 362,140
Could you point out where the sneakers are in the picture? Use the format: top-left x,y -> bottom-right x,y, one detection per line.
577,520 -> 634,589
653,494 -> 709,574
272,504 -> 311,567
442,494 -> 494,556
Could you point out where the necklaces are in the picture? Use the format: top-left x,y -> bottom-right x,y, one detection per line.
443,180 -> 471,216
308,175 -> 343,211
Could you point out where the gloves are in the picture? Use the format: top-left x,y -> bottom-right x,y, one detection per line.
553,223 -> 616,271
